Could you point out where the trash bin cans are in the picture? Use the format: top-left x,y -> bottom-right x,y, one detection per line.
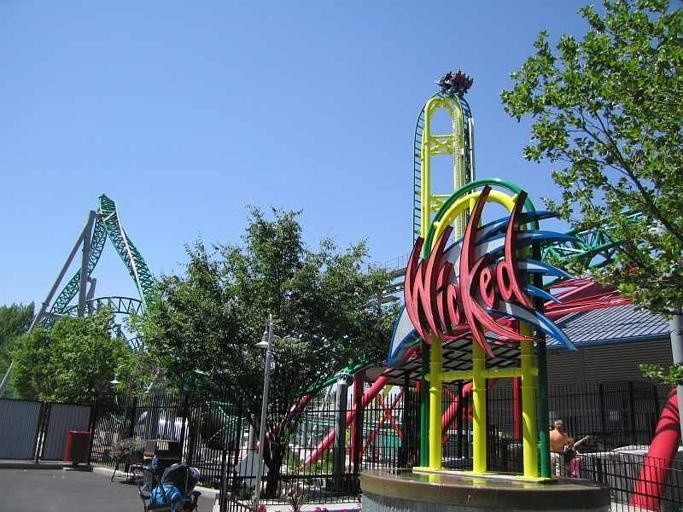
63,431 -> 90,471
143,440 -> 181,491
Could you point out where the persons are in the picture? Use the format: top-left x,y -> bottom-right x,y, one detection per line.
550,419 -> 569,477
564,448 -> 583,478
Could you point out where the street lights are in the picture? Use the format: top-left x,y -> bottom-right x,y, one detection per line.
248,311 -> 274,512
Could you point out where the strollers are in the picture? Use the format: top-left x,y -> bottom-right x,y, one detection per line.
128,462 -> 205,512
